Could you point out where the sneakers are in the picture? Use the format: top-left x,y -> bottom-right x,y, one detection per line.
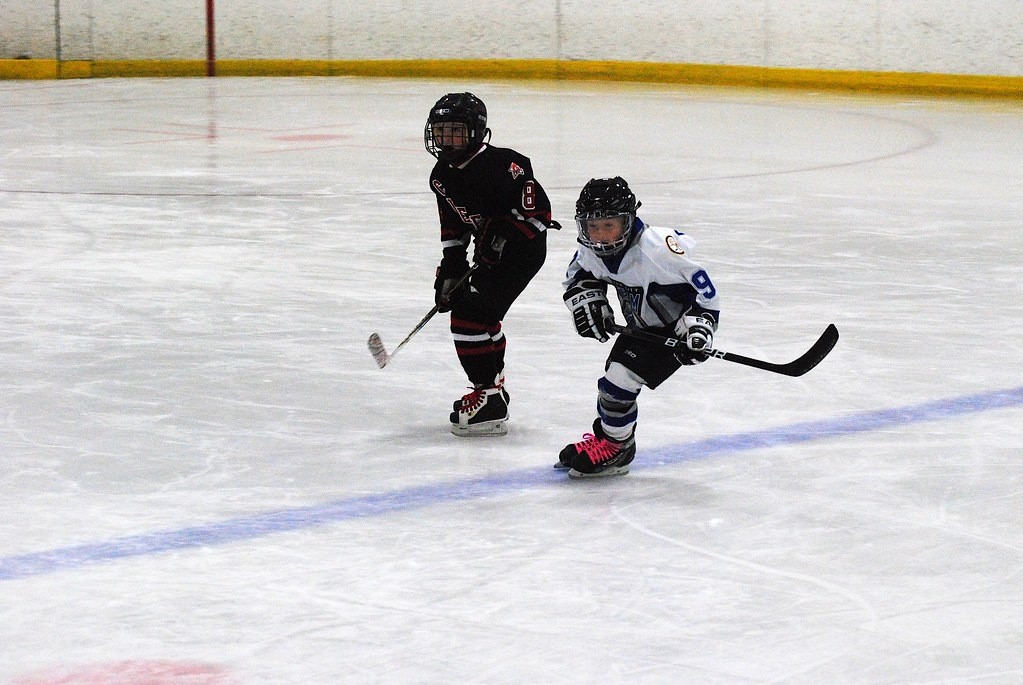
568,422 -> 637,479
553,417 -> 601,469
453,368 -> 510,411
450,372 -> 509,437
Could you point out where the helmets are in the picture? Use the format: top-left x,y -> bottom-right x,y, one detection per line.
424,92 -> 488,166
575,176 -> 641,256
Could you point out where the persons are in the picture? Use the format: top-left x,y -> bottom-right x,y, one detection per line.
422,91 -> 563,436
554,176 -> 720,480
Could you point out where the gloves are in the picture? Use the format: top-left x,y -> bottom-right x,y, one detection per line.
480,233 -> 511,270
563,280 -> 616,343
673,305 -> 717,365
435,259 -> 471,313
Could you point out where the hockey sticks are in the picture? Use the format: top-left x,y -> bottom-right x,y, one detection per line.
608,322 -> 840,378
366,232 -> 508,370
